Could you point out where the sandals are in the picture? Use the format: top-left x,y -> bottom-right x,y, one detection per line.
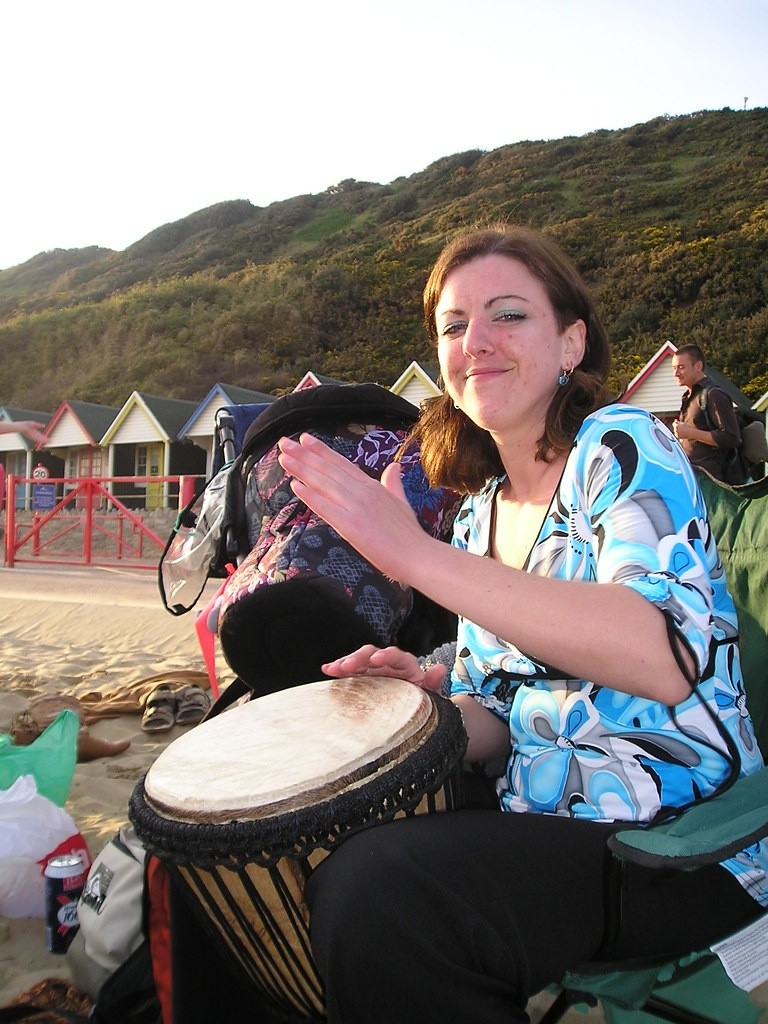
175,683 -> 209,725
141,683 -> 174,732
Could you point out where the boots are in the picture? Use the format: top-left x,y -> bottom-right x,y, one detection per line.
9,693 -> 131,764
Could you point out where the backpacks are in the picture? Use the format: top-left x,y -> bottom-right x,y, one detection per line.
701,383 -> 768,486
217,427 -> 431,695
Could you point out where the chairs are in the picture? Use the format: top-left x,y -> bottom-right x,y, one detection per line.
209,402 -> 270,566
540,463 -> 768,1024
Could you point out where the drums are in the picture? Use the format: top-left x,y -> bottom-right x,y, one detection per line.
126,675 -> 470,1024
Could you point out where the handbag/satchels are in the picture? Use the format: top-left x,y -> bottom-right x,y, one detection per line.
75,823 -> 150,972
0,709 -> 92,918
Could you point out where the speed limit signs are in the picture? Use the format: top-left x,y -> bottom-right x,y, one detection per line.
32,466 -> 48,480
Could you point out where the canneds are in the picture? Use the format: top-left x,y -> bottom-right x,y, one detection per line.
43,855 -> 86,954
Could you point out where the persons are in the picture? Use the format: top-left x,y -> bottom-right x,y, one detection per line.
0,420 -> 50,445
277,225 -> 768,1024
672,344 -> 739,485
